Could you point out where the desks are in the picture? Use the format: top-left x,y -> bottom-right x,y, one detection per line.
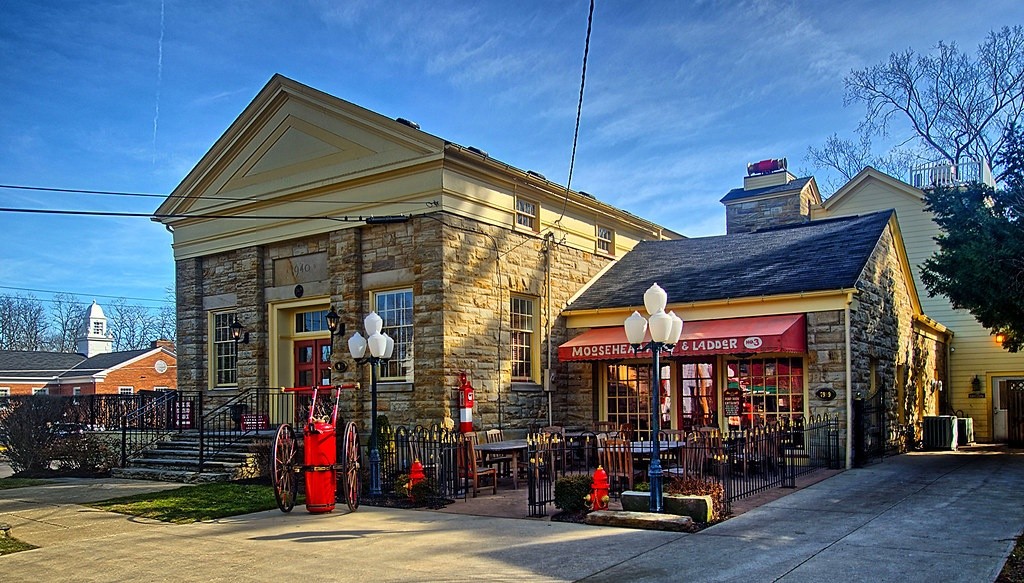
598,441 -> 686,480
559,431 -> 618,471
472,439 -> 549,491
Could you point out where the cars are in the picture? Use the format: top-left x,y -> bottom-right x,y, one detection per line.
0,423 -> 105,460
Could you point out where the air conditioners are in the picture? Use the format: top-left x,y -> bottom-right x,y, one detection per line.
957,418 -> 974,443
921,416 -> 958,450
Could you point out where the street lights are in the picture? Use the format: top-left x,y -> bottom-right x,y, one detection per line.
623,283 -> 685,514
347,311 -> 396,499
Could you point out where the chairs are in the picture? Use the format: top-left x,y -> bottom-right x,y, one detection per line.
450,420 -> 785,498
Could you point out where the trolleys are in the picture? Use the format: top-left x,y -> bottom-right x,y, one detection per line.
268,382 -> 363,513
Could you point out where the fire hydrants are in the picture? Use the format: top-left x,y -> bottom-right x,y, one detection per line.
583,465 -> 610,511
402,457 -> 426,503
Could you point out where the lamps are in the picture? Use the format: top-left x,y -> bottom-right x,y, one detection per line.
229,317 -> 249,345
324,305 -> 345,336
971,375 -> 980,391
996,332 -> 1007,343
